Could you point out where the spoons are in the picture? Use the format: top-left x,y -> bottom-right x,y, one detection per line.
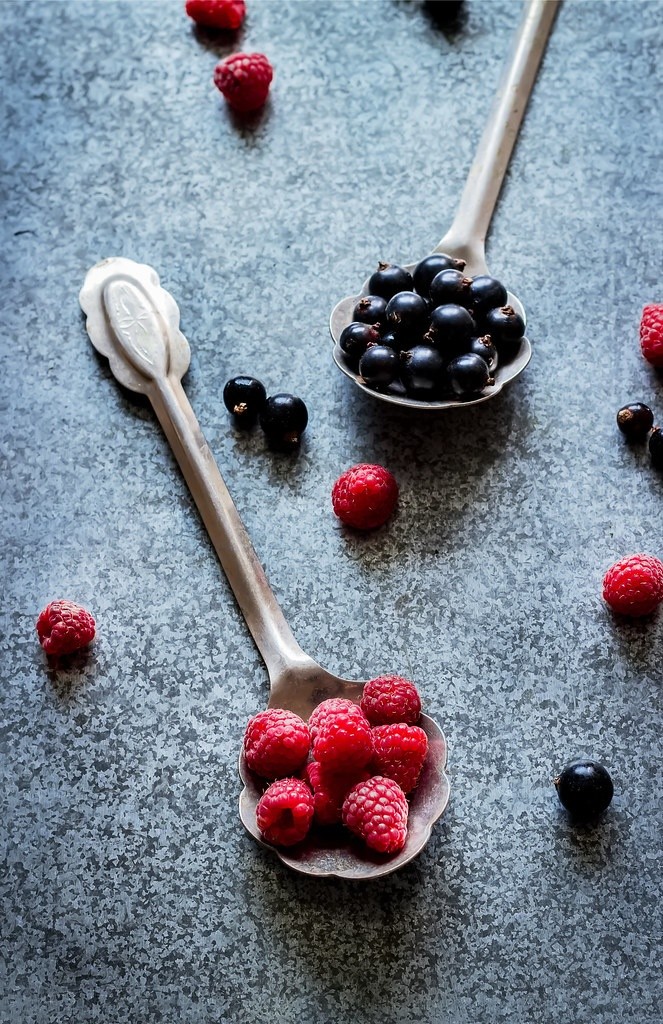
330,1 -> 564,409
79,256 -> 449,879
